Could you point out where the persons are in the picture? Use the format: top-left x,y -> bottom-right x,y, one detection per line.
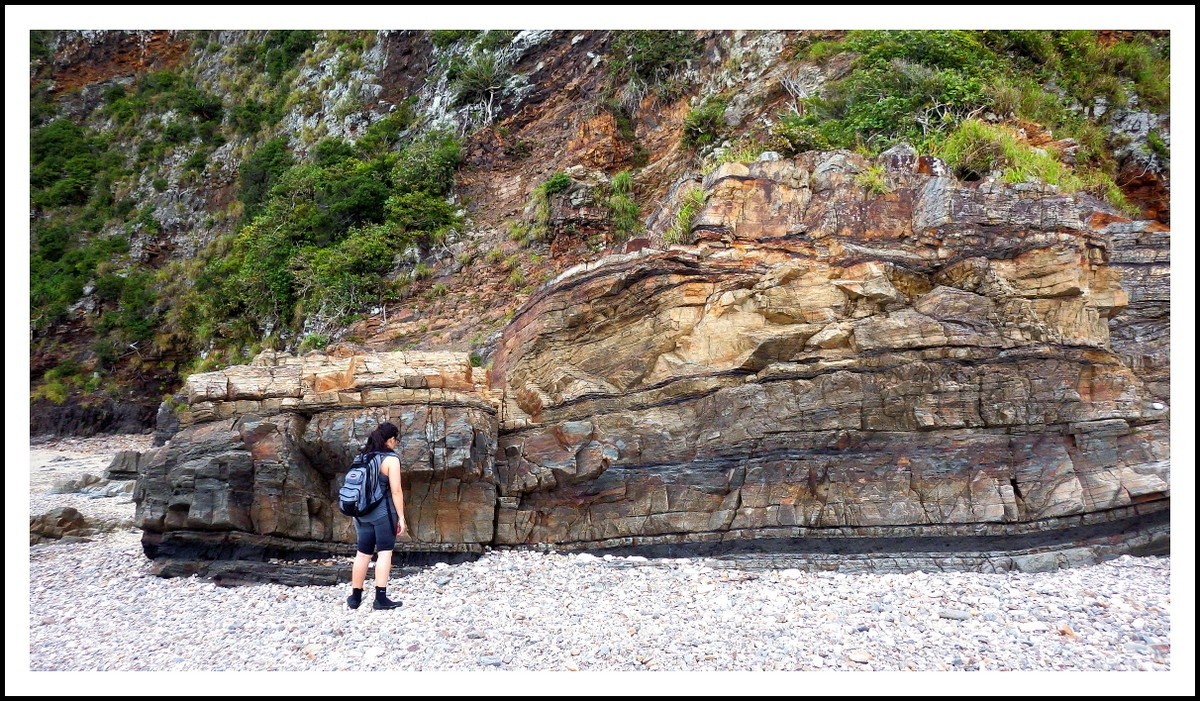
347,422 -> 408,609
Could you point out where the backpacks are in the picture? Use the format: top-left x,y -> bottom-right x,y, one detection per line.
337,451 -> 402,517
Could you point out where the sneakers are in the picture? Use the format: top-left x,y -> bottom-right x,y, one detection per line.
373,597 -> 402,610
347,595 -> 360,609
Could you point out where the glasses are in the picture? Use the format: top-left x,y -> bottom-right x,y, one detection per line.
396,439 -> 401,444
